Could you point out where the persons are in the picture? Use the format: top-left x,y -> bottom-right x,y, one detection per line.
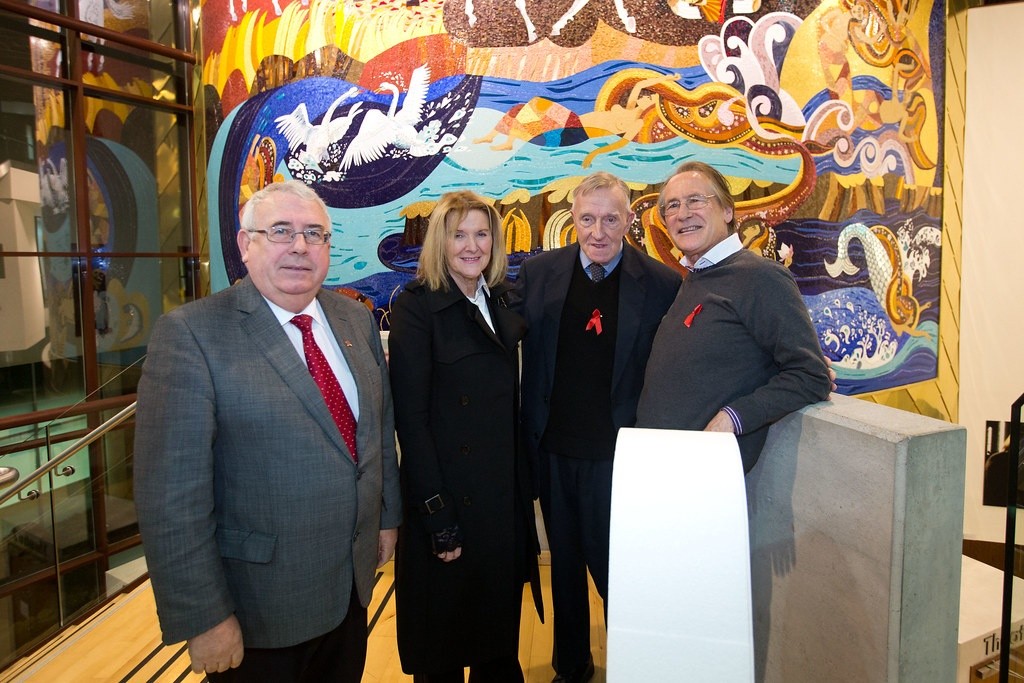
389,189 -> 546,683
633,161 -> 830,477
504,172 -> 838,683
132,181 -> 403,683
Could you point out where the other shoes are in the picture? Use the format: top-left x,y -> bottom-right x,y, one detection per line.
551,652 -> 594,683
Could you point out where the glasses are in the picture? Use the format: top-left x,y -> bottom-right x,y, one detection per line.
659,195 -> 716,216
248,226 -> 331,245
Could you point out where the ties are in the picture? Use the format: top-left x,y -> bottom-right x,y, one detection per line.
290,315 -> 358,467
588,263 -> 604,285
